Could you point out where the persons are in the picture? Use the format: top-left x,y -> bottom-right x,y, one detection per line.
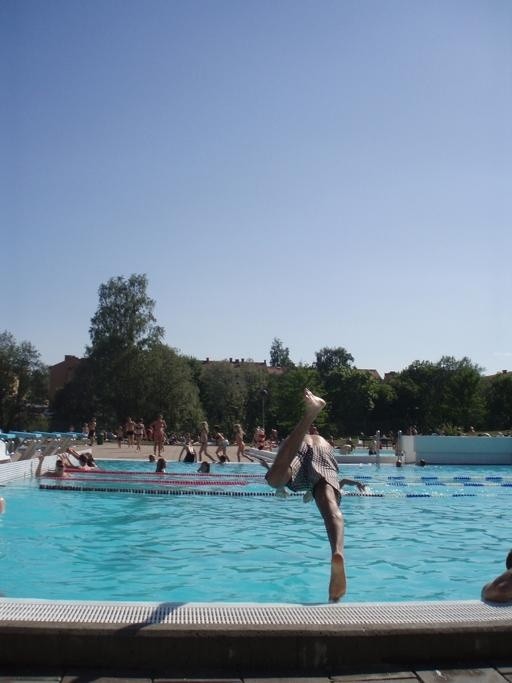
57,448 -> 98,471
156,459 -> 167,473
68,417 -> 108,444
199,421 -> 217,462
482,548 -> 512,601
149,454 -> 155,462
265,388 -> 347,602
217,455 -> 227,464
35,456 -> 72,477
118,417 -> 279,452
149,414 -> 167,456
339,478 -> 365,492
198,461 -> 210,474
369,425 -> 425,467
214,425 -> 230,462
178,440 -> 197,463
233,424 -> 254,462
309,424 -> 318,435
